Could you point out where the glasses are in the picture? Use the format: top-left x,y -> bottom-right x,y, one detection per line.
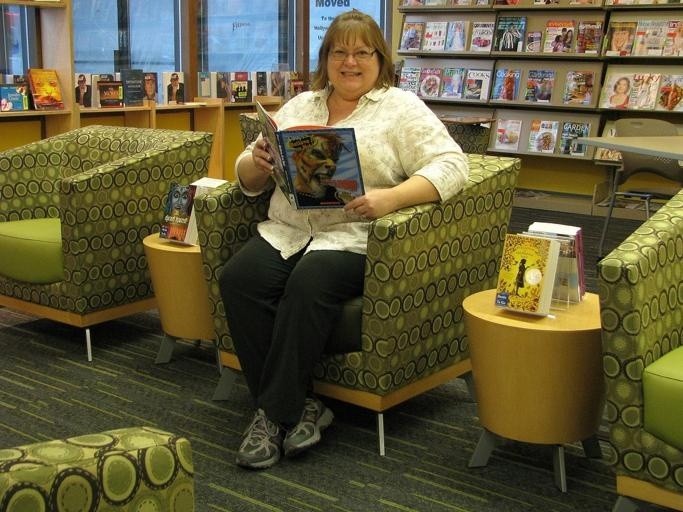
331,45 -> 377,61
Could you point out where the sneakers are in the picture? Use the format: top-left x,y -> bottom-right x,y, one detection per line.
235,401 -> 297,470
284,398 -> 335,458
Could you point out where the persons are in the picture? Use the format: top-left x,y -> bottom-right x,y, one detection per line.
217,8 -> 468,470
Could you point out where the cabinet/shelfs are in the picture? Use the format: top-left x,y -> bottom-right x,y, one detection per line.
383,0 -> 682,221
0,1 -> 81,157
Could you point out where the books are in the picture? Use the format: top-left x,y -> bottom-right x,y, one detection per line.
254,100 -> 365,211
493,220 -> 585,317
158,177 -> 227,246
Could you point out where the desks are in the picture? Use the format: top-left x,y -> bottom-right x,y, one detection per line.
573,132 -> 682,259
139,230 -> 218,370
458,283 -> 609,494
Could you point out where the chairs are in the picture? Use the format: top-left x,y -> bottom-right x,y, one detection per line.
192,139 -> 522,459
1,427 -> 201,512
595,183 -> 683,512
2,123 -> 213,367
599,116 -> 682,255
